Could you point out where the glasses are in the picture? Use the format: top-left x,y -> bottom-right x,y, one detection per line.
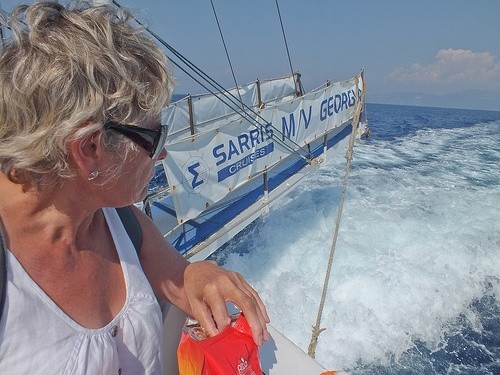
103,120 -> 168,159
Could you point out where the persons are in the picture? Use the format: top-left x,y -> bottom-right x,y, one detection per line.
1,0 -> 271,375
